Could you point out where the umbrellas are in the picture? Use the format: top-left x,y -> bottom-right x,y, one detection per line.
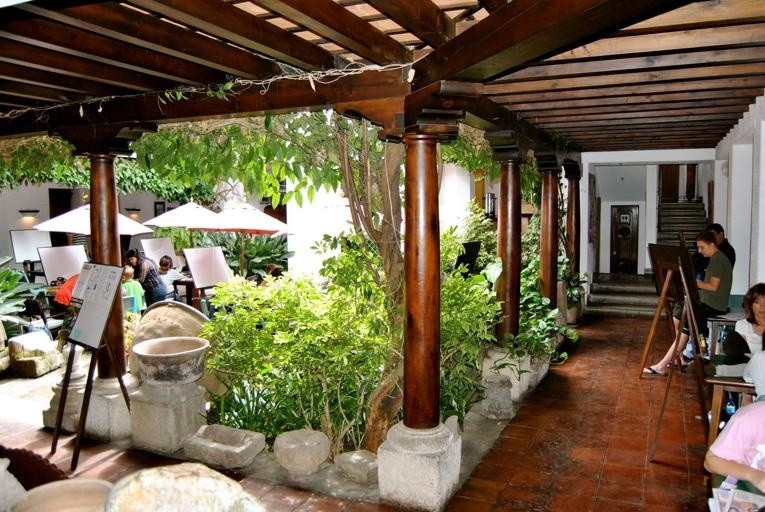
142,202 -> 295,277
33,203 -> 154,235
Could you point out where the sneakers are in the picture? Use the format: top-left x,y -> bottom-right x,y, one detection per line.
683,355 -> 694,365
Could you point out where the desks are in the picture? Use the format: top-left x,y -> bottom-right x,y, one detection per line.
701,355 -> 758,449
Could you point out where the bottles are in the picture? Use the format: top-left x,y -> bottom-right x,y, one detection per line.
726,399 -> 735,416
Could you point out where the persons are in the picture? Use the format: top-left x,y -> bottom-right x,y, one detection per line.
724,283 -> 765,365
47,274 -> 80,320
121,248 -> 184,317
704,329 -> 765,493
643,223 -> 736,375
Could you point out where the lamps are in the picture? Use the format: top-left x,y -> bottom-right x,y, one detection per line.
125,208 -> 141,220
17,209 -> 40,226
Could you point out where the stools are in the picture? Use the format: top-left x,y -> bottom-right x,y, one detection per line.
707,317 -> 737,357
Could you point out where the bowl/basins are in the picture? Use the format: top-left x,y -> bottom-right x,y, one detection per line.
132,336 -> 210,386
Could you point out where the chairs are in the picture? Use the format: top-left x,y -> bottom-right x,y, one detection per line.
15,299 -> 63,341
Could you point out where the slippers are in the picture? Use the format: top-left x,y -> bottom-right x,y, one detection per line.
643,367 -> 667,376
666,362 -> 688,368
